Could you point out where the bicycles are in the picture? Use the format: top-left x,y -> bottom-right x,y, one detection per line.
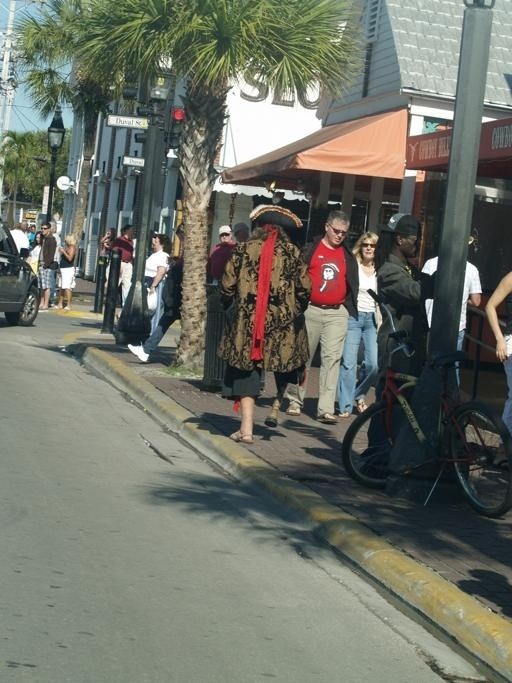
340,288 -> 512,518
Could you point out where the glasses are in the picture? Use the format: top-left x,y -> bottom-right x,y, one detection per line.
329,223 -> 348,236
362,242 -> 377,247
407,237 -> 420,245
41,227 -> 48,229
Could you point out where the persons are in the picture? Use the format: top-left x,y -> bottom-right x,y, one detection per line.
282,210 -> 360,423
128,256 -> 182,362
0,220 -> 77,310
366,214 -> 480,446
217,204 -> 314,444
209,225 -> 236,282
99,224 -> 135,314
339,232 -> 384,418
484,271 -> 512,436
144,232 -> 173,335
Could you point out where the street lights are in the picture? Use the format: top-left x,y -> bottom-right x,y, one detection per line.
47,104 -> 66,223
113,51 -> 176,346
11,154 -> 46,225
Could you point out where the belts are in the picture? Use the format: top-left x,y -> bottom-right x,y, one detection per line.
310,302 -> 339,309
122,260 -> 129,263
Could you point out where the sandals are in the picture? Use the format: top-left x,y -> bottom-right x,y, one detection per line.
229,430 -> 256,442
339,411 -> 350,417
285,404 -> 301,415
316,411 -> 336,422
356,398 -> 368,413
265,409 -> 278,428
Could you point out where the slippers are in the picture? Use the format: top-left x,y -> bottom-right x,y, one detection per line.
491,458 -> 509,469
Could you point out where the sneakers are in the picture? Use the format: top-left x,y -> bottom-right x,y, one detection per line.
37,305 -> 71,312
128,343 -> 149,361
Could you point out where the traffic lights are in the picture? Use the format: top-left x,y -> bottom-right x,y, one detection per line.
168,106 -> 184,147
121,46 -> 143,104
134,104 -> 161,144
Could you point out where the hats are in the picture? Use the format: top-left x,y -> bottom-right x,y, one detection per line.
230,222 -> 249,232
249,203 -> 304,231
375,212 -> 421,235
218,225 -> 232,235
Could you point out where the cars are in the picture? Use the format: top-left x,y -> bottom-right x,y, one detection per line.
0,216 -> 41,326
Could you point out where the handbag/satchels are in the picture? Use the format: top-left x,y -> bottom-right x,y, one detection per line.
146,287 -> 157,310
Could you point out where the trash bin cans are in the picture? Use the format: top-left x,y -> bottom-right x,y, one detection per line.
203,282 -> 240,392
